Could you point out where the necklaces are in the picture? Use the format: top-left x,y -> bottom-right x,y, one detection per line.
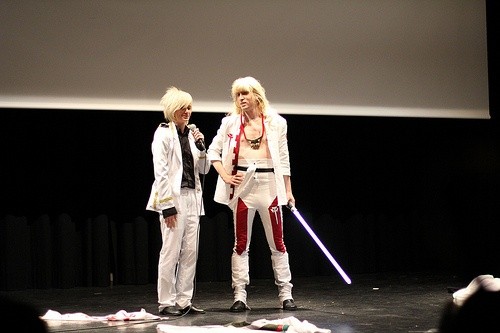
241,113 -> 265,150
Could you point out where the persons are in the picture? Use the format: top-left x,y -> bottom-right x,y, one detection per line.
146,86 -> 212,317
207,76 -> 298,312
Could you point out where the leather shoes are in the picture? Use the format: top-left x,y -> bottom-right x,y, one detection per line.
159,306 -> 181,315
231,300 -> 246,311
179,304 -> 205,314
283,298 -> 297,309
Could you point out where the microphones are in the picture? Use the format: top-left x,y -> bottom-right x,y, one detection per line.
190,124 -> 205,150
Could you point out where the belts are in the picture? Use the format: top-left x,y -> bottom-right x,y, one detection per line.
237,166 -> 274,171
